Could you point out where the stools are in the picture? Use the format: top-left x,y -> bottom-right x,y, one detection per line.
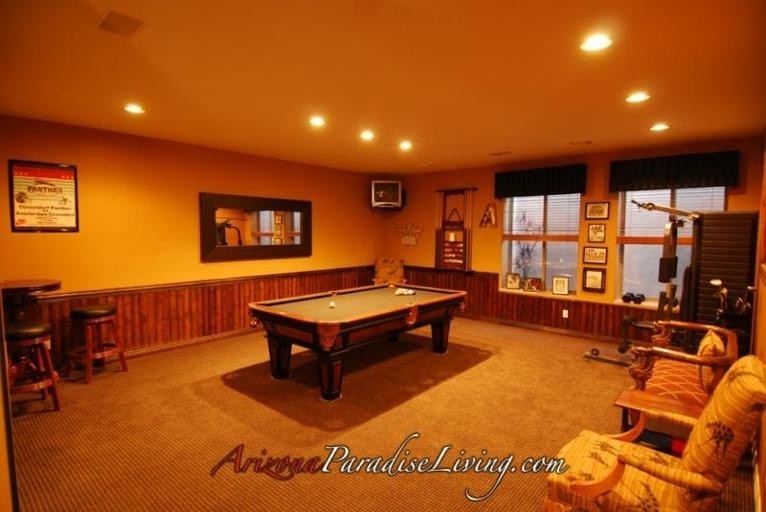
68,304 -> 129,385
5,321 -> 64,413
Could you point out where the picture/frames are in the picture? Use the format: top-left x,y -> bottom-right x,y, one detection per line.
588,223 -> 606,243
585,202 -> 610,220
7,160 -> 80,233
524,277 -> 542,292
582,266 -> 607,294
506,272 -> 521,289
552,276 -> 570,295
583,246 -> 608,265
272,213 -> 284,246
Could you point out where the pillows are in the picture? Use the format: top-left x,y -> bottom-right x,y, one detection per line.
697,328 -> 726,397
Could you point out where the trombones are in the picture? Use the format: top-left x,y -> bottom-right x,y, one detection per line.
330,303 -> 335,308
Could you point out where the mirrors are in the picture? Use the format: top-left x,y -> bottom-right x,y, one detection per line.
196,191 -> 314,264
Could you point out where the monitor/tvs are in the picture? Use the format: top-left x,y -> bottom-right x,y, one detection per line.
371,181 -> 402,208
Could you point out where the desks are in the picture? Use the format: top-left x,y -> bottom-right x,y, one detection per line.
2,279 -> 60,387
248,281 -> 467,403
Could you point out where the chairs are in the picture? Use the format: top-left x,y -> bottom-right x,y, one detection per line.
371,254 -> 408,285
546,352 -> 766,511
613,320 -> 742,437
